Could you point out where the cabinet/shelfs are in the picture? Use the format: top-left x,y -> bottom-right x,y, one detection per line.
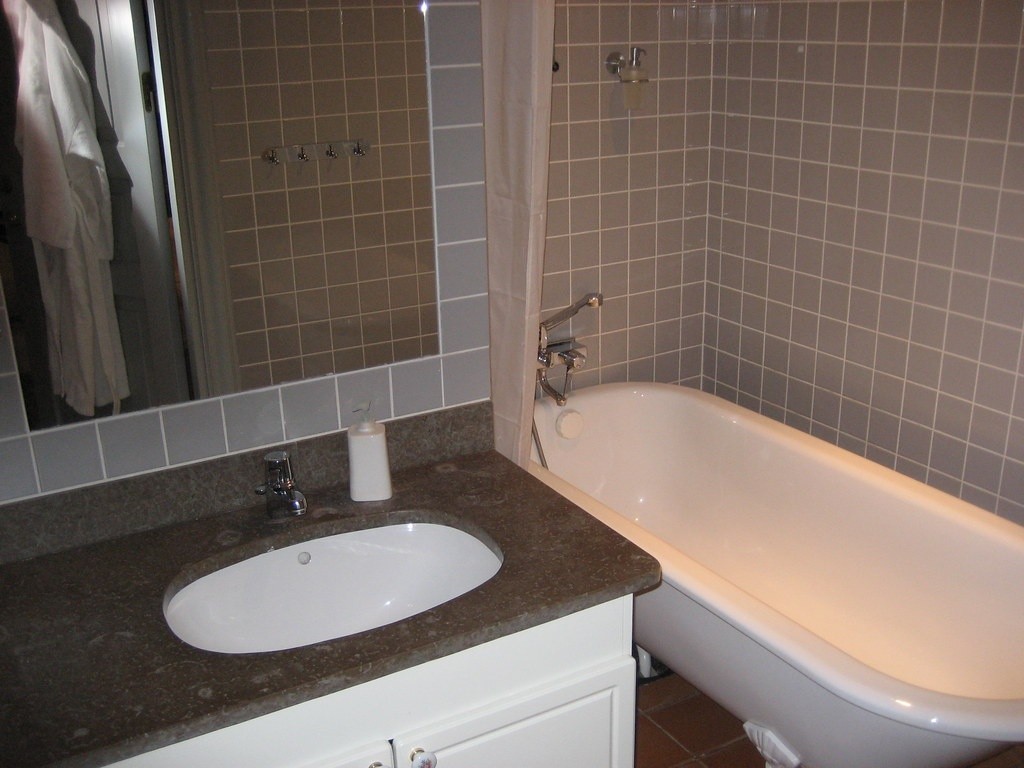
92,592 -> 640,768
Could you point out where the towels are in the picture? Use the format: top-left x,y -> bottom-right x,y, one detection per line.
1,1 -> 130,418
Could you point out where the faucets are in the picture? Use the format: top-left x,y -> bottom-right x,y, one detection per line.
254,449 -> 306,519
540,291 -> 605,350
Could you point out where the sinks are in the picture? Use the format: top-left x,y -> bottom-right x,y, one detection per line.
163,509 -> 508,654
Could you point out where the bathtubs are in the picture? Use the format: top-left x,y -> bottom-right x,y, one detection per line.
531,381 -> 1023,767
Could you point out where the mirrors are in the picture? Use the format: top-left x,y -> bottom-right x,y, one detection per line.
0,1 -> 441,436
